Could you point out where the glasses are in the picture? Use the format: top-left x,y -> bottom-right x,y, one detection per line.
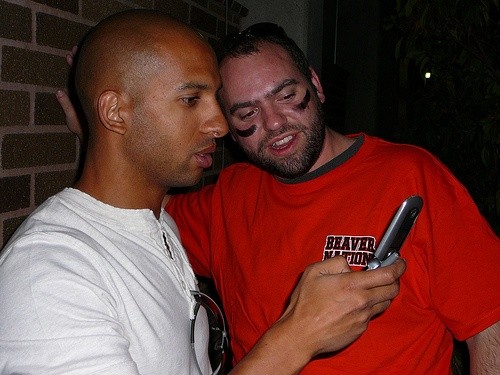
191,291 -> 233,375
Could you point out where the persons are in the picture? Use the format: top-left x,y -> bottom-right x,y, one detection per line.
56,23 -> 500,375
1,9 -> 406,375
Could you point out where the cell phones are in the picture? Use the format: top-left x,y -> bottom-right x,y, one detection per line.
361,195 -> 424,273
